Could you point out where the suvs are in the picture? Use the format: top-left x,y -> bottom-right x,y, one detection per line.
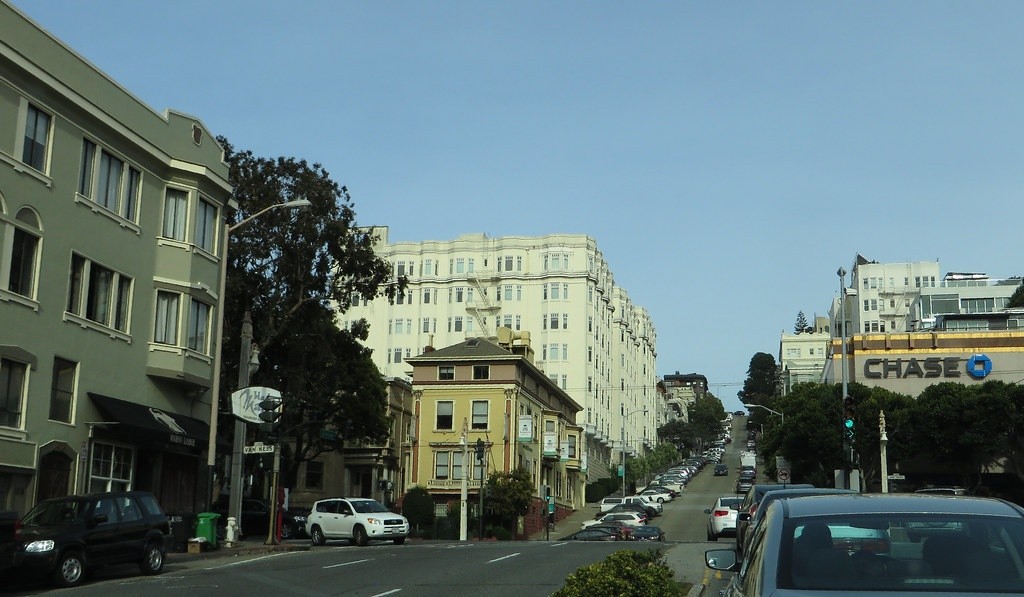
306,497 -> 409,547
905,486 -> 990,545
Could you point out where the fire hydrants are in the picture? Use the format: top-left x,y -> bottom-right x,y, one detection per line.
224,517 -> 239,543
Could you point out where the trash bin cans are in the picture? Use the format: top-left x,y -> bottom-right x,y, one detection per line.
166,512 -> 196,553
196,513 -> 221,550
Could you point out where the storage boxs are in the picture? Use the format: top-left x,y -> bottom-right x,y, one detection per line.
188,541 -> 206,553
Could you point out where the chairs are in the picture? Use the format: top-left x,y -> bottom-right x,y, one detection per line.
926,535 -> 982,576
968,552 -> 1020,583
793,536 -> 834,576
803,549 -> 858,580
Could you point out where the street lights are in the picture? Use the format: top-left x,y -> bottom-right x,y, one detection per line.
717,385 -> 727,397
459,416 -> 470,541
204,196 -> 314,513
227,309 -> 262,533
744,404 -> 784,427
622,409 -> 649,498
877,410 -> 889,493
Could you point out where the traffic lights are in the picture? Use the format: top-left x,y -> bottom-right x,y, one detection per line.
546,487 -> 550,501
842,396 -> 856,440
259,401 -> 284,434
477,438 -> 484,460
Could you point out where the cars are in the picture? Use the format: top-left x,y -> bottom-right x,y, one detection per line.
735,483 -> 893,559
556,411 -> 758,543
216,496 -> 312,540
0,491 -> 174,587
703,497 -> 745,542
705,492 -> 1024,597
